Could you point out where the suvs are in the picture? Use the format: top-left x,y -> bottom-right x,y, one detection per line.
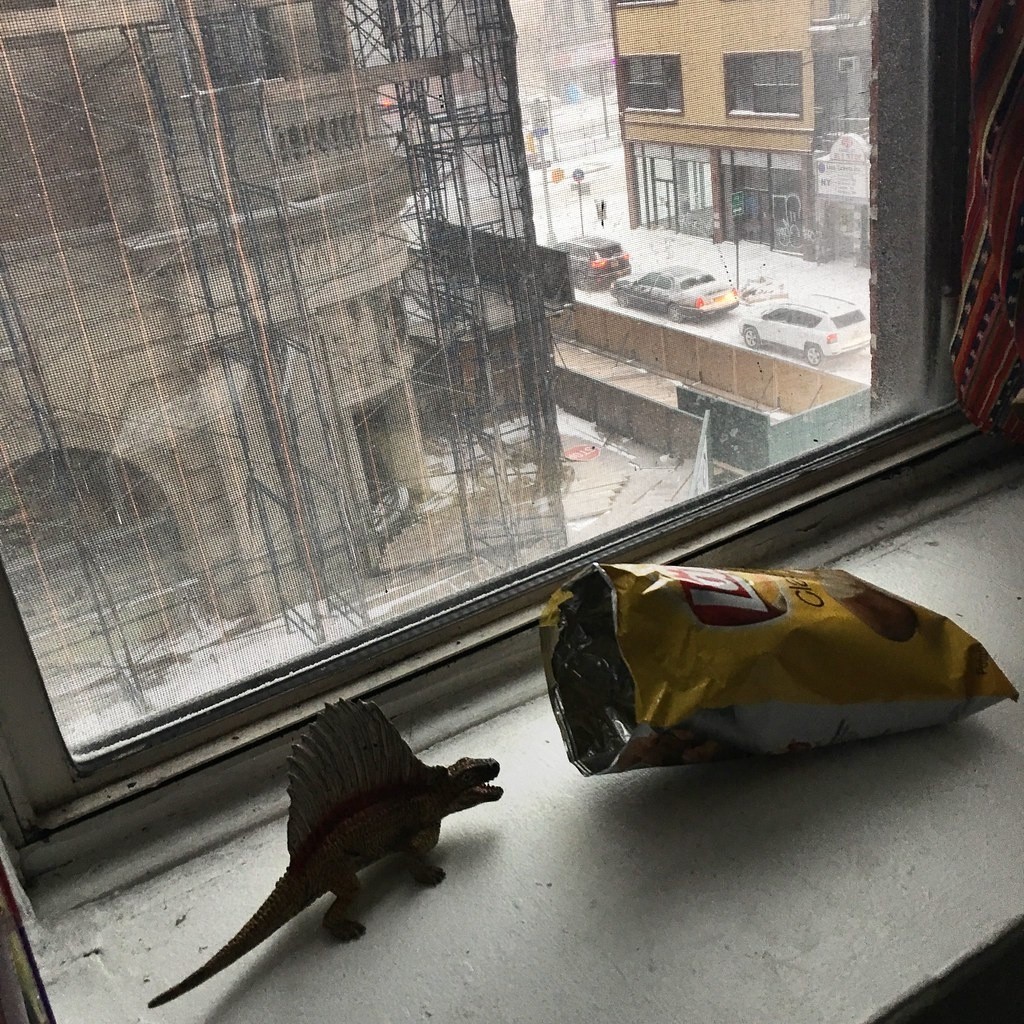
547,235 -> 632,292
740,293 -> 871,368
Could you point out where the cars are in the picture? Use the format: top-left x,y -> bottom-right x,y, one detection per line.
609,265 -> 740,324
